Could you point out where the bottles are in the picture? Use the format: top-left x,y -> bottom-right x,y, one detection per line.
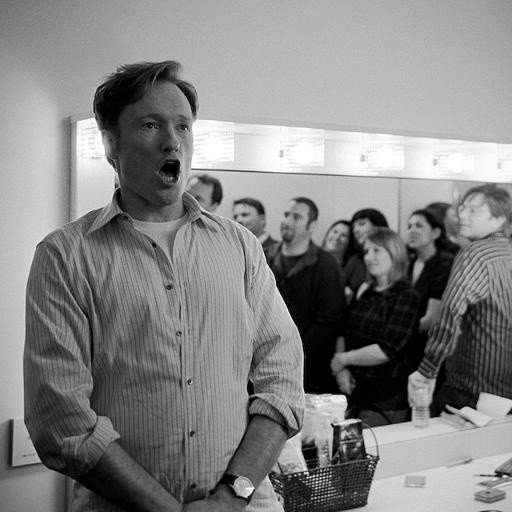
411,378 -> 430,428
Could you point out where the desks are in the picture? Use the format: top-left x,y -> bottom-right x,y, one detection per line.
334,415 -> 512,511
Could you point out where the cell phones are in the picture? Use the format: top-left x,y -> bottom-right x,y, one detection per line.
481,477 -> 512,488
405,475 -> 426,487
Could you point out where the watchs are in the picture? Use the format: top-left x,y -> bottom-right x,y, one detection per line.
219,471 -> 257,504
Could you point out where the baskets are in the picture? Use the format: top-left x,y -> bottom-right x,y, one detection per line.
270,442 -> 380,511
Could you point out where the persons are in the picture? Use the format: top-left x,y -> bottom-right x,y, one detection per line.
21,58 -> 306,510
321,183 -> 512,430
185,171 -> 346,393
